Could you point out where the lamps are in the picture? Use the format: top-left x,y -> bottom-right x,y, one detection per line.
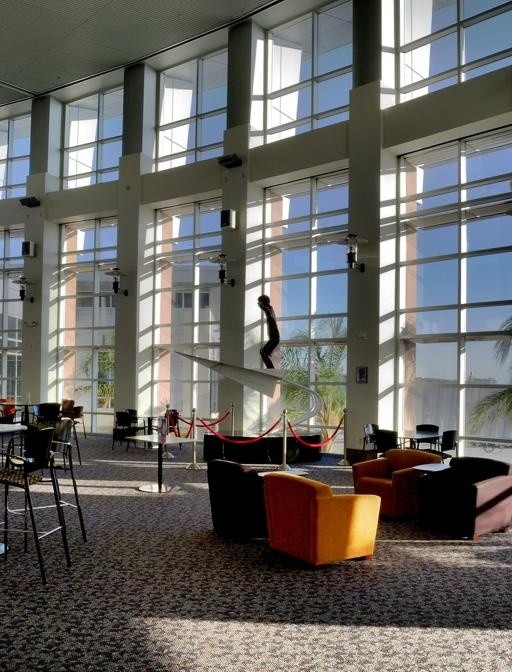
336,232 -> 369,275
209,253 -> 235,289
12,276 -> 35,303
104,267 -> 129,297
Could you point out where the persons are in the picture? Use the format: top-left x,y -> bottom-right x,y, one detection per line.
257,295 -> 280,369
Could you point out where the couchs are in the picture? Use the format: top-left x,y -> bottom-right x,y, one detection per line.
264,471 -> 381,566
208,458 -> 268,547
351,448 -> 441,520
417,456 -> 511,543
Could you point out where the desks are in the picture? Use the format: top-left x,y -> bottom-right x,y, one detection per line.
412,463 -> 452,527
241,463 -> 310,479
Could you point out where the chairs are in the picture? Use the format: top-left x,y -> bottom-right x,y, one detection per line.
1,397 -> 88,586
110,408 -> 182,453
361,422 -> 459,459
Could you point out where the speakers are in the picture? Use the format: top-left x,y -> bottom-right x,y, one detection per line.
22,241 -> 34,257
220,209 -> 236,231
